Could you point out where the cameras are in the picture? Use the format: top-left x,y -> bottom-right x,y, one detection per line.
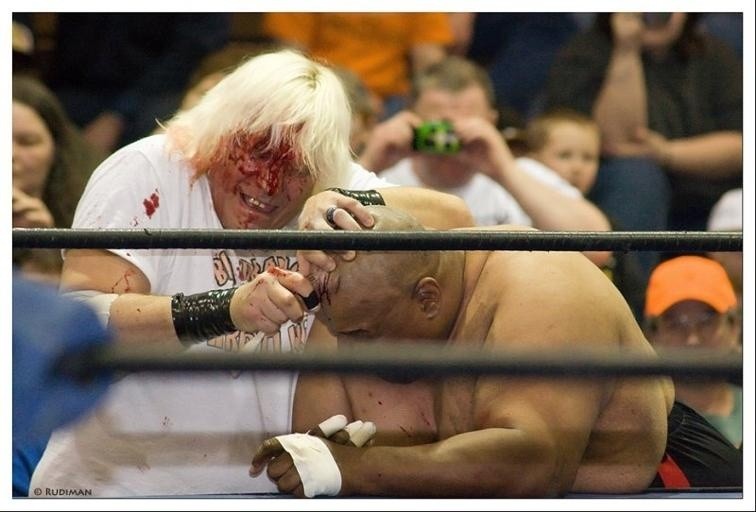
411,123 -> 464,153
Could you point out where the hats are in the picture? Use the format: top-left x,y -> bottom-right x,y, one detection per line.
641,253 -> 737,318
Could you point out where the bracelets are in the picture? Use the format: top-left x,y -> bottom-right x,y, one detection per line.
170,289 -> 239,350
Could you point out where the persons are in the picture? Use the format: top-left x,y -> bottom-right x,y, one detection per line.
12,13 -> 742,330
247,202 -> 742,499
25,47 -> 479,500
629,253 -> 743,493
